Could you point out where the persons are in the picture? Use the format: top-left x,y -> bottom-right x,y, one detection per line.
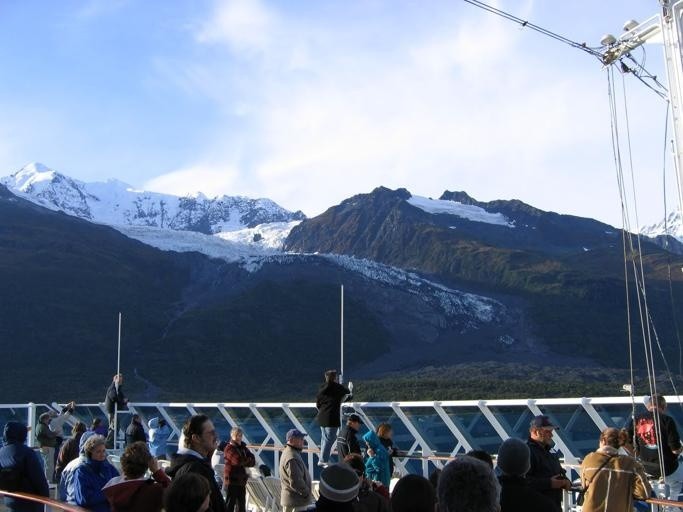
103,371 -> 130,431
313,368 -> 353,466
0,394 -> 683,512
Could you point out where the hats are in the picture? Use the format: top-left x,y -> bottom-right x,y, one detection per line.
530,416 -> 552,428
320,465 -> 359,502
496,439 -> 530,476
390,475 -> 435,512
347,415 -> 364,424
285,429 -> 308,441
344,453 -> 364,476
438,456 -> 499,512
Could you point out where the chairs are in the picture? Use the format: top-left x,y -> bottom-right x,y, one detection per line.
106,454 -> 320,512
